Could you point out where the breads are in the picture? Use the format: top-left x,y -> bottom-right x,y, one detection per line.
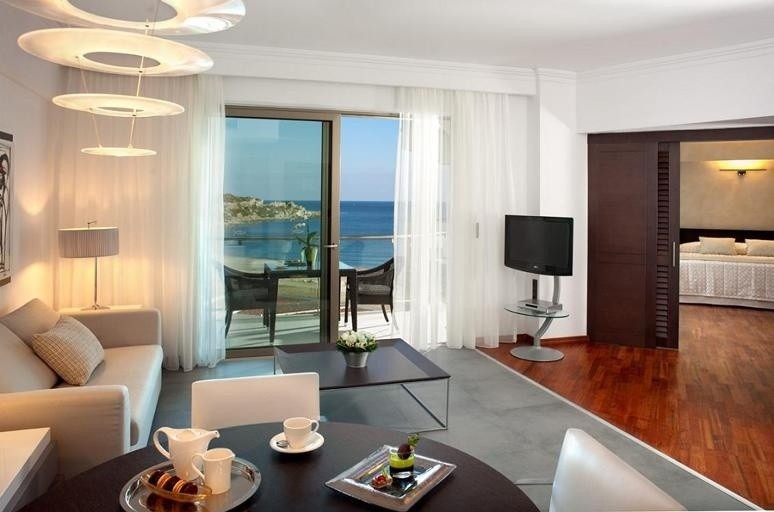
149,470 -> 197,495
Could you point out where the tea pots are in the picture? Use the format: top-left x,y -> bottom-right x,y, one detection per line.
152,425 -> 220,480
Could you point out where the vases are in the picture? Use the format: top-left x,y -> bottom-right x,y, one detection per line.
342,352 -> 369,369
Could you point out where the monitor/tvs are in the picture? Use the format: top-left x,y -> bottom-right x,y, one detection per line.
503,214 -> 574,277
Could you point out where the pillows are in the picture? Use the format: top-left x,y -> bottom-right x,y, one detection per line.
30,314 -> 105,386
698,235 -> 738,256
0,321 -> 59,393
679,241 -> 747,254
744,238 -> 774,256
0,297 -> 62,372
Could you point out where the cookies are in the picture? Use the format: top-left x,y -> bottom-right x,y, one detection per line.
146,493 -> 197,511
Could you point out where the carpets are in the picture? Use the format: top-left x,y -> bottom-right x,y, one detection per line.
144,341 -> 766,511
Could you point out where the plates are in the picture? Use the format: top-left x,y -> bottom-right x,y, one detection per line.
119,454 -> 262,511
323,445 -> 457,512
269,430 -> 325,455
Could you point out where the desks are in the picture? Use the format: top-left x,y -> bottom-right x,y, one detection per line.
503,298 -> 570,362
0,427 -> 59,512
13,422 -> 543,512
264,260 -> 358,342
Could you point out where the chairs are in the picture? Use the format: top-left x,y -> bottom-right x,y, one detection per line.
345,255 -> 406,333
515,427 -> 687,512
190,371 -> 321,431
214,261 -> 278,338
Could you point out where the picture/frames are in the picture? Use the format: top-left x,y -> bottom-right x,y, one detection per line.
0,130 -> 14,287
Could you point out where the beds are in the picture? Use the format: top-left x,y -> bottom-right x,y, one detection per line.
678,227 -> 774,310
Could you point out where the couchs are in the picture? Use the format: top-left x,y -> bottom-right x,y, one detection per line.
0,308 -> 164,476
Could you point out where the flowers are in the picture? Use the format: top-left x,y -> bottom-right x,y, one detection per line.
335,329 -> 379,353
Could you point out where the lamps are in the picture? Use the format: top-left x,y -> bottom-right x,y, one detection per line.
0,1 -> 247,159
57,218 -> 120,310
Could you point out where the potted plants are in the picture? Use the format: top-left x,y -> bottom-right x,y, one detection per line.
296,223 -> 321,267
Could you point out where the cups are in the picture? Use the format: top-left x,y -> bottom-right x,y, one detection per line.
190,447 -> 236,495
388,444 -> 415,480
282,416 -> 320,450
